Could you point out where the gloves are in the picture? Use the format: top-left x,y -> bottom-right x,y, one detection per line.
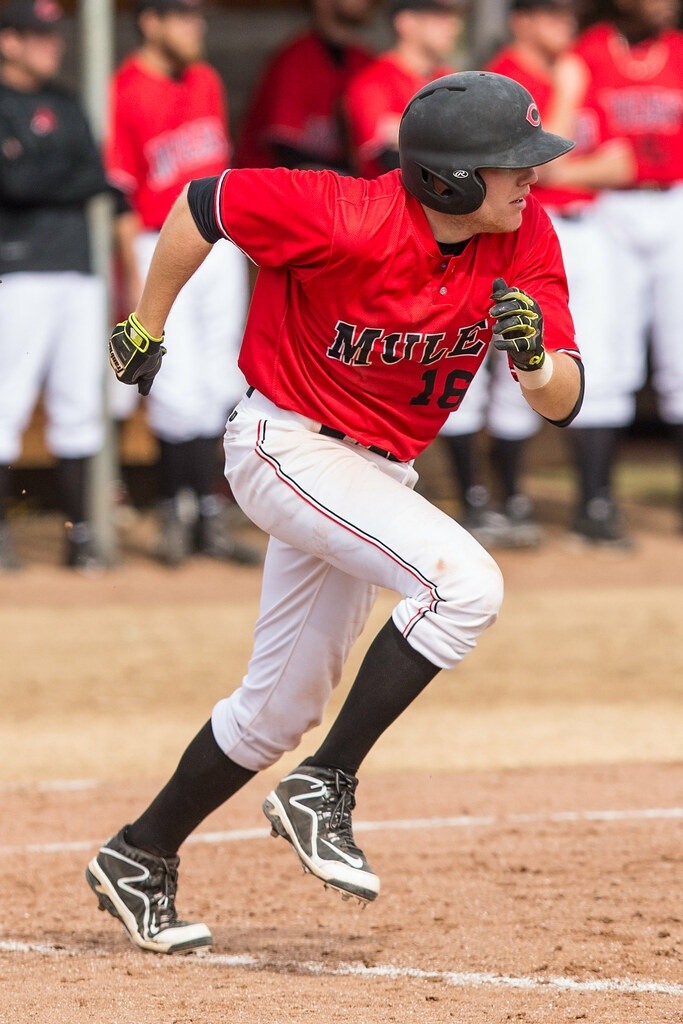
109,311 -> 166,397
489,278 -> 545,371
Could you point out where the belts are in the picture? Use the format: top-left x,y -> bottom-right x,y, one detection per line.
246,385 -> 400,463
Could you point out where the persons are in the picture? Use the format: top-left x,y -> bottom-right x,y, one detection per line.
231,0 -> 374,173
344,0 -> 683,558
103,0 -> 262,563
88,70 -> 584,957
0,0 -> 131,573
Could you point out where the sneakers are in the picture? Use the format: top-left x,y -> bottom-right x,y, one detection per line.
86,824 -> 212,955
263,756 -> 381,904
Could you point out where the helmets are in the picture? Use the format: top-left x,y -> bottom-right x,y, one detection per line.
399,70 -> 576,214
